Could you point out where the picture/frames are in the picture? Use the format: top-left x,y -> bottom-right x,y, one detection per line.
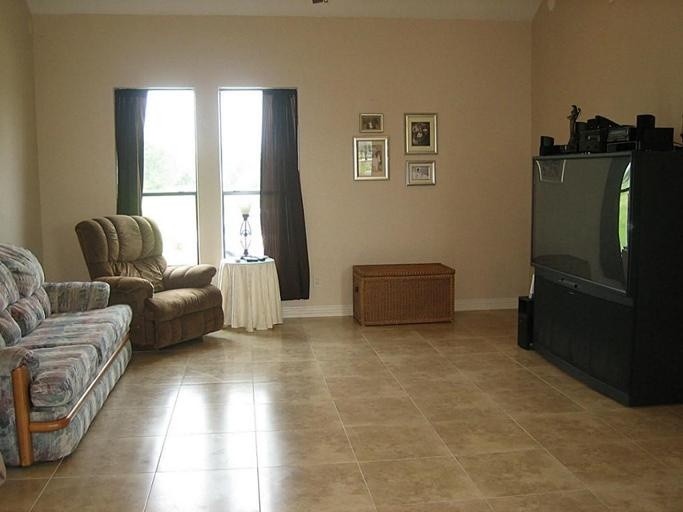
352,137 -> 391,181
405,112 -> 438,156
358,111 -> 384,133
404,159 -> 435,186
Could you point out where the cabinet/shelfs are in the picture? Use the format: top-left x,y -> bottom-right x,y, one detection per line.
528,278 -> 683,408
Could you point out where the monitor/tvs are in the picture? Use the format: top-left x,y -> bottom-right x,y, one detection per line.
529,151 -> 638,310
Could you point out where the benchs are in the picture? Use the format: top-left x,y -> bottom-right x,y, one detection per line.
352,261 -> 457,328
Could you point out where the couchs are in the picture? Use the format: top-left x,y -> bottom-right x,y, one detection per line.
0,243 -> 134,468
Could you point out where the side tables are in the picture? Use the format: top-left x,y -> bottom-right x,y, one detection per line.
210,258 -> 283,331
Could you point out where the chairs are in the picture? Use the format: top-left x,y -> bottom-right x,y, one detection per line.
74,214 -> 224,354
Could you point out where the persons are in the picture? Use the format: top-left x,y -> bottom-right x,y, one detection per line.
415,124 -> 428,145
415,168 -> 424,178
366,120 -> 376,129
370,145 -> 382,175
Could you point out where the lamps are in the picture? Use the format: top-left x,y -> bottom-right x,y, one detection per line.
239,205 -> 250,256
